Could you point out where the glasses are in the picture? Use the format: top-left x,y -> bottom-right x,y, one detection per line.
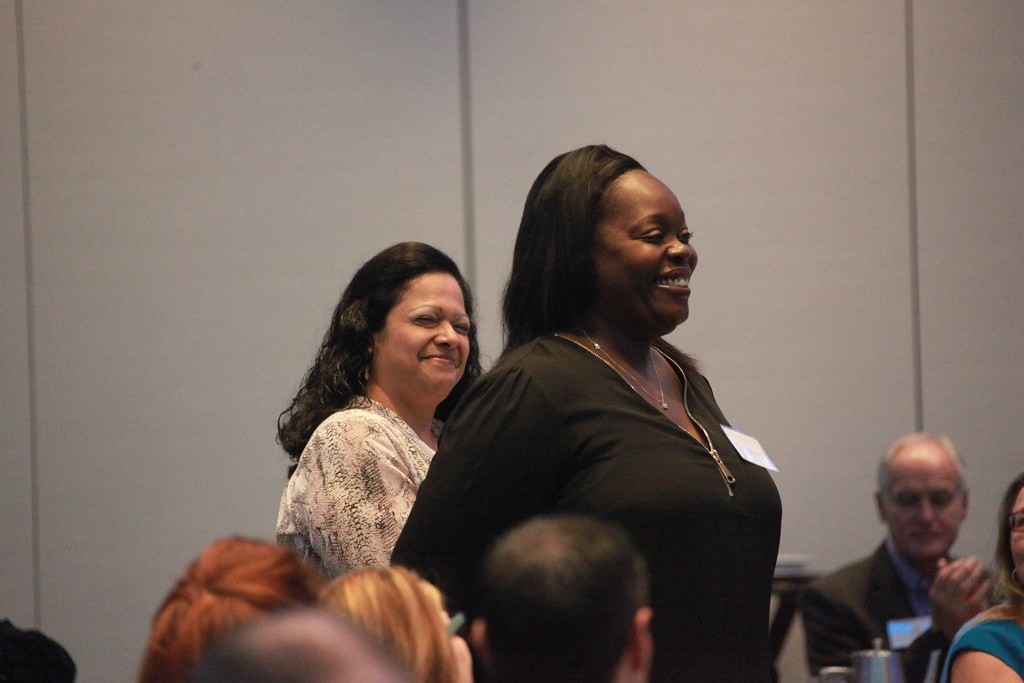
1008,511 -> 1024,531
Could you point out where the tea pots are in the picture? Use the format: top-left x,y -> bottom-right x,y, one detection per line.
820,637 -> 941,683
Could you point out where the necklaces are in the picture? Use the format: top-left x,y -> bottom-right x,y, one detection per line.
579,324 -> 668,408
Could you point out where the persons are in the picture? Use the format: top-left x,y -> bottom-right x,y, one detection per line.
389,145 -> 783,683
316,567 -> 471,683
276,242 -> 482,577
0,618 -> 77,683
473,518 -> 653,683
803,435 -> 992,683
140,536 -> 315,683
948,474 -> 1024,683
195,612 -> 399,683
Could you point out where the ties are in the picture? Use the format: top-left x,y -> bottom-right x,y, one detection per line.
917,577 -> 934,617
